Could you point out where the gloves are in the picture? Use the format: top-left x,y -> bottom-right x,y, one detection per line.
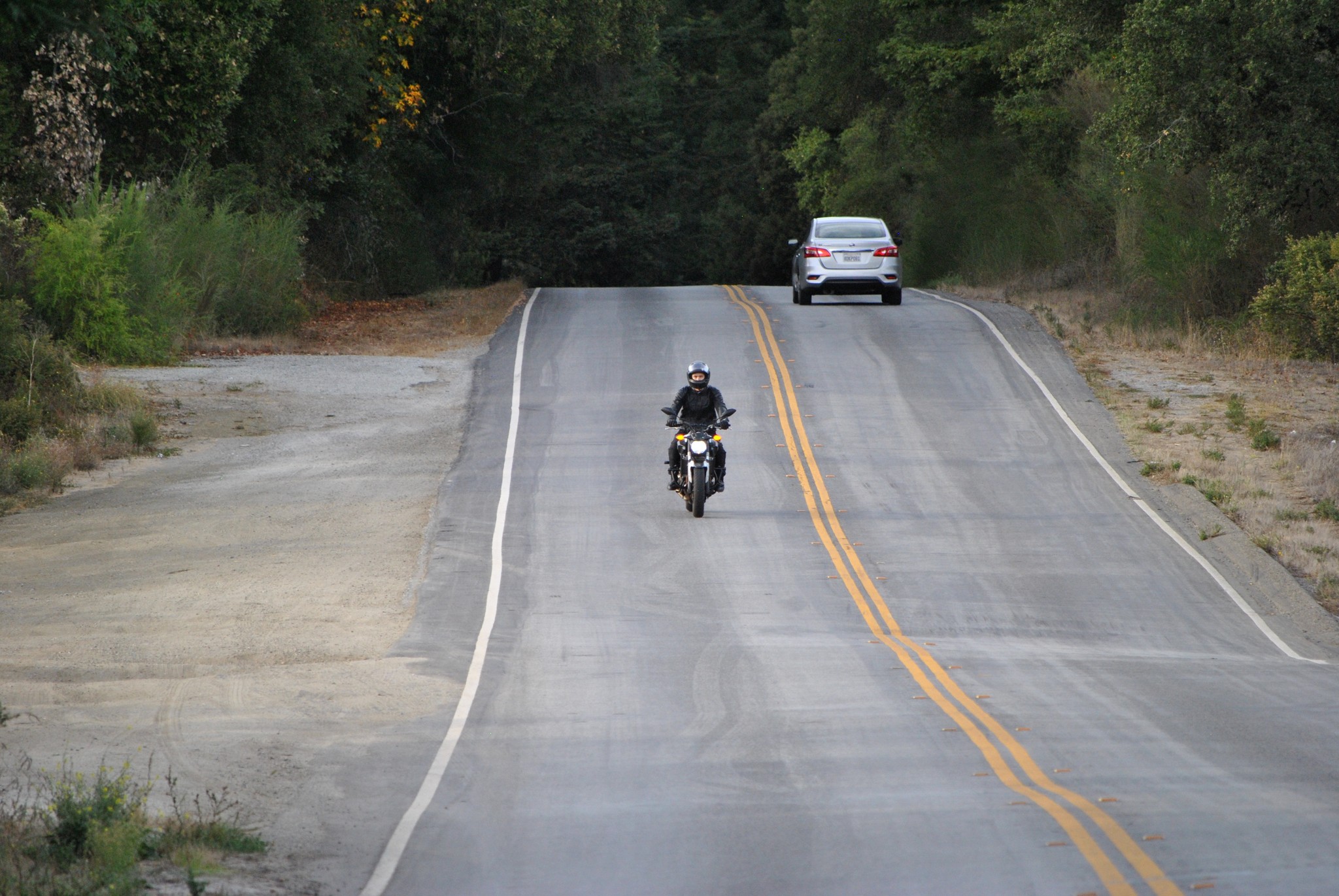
720,418 -> 729,430
667,419 -> 677,427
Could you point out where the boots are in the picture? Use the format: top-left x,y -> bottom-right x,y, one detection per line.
667,468 -> 679,490
713,467 -> 726,492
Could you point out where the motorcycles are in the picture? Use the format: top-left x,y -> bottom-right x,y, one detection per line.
660,406 -> 737,518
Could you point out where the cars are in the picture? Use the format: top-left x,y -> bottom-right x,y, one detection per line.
788,215 -> 903,305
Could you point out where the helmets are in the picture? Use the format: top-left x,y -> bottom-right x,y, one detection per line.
687,361 -> 710,389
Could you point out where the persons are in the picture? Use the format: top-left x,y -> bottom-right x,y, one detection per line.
664,361 -> 730,492
